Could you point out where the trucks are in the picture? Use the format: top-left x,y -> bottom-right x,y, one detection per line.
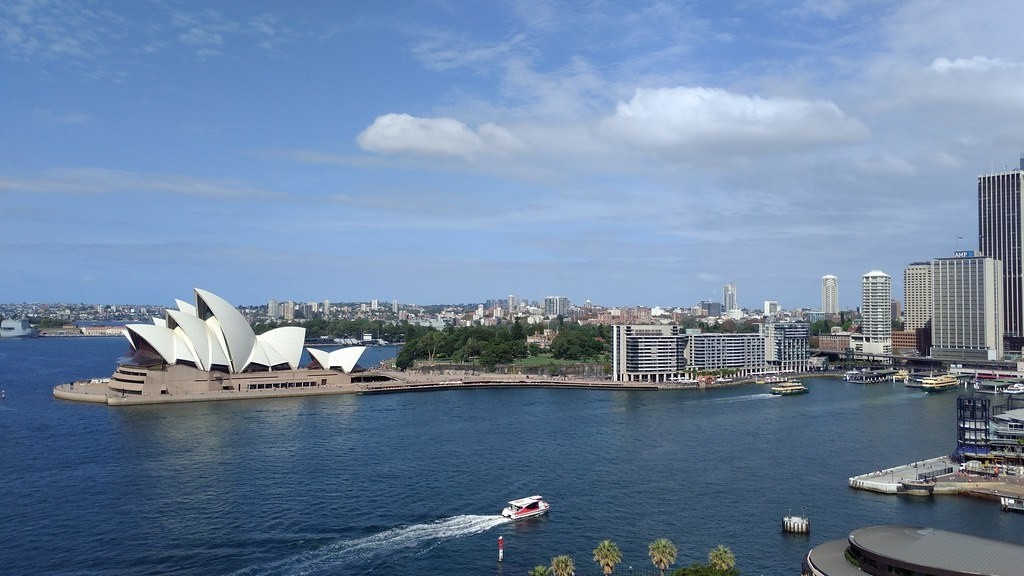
958,461 -> 998,477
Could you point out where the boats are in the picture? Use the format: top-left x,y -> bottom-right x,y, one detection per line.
501,495 -> 550,520
843,368 -> 860,381
921,369 -> 957,392
894,370 -> 908,381
769,379 -> 809,396
1002,384 -> 1024,394
